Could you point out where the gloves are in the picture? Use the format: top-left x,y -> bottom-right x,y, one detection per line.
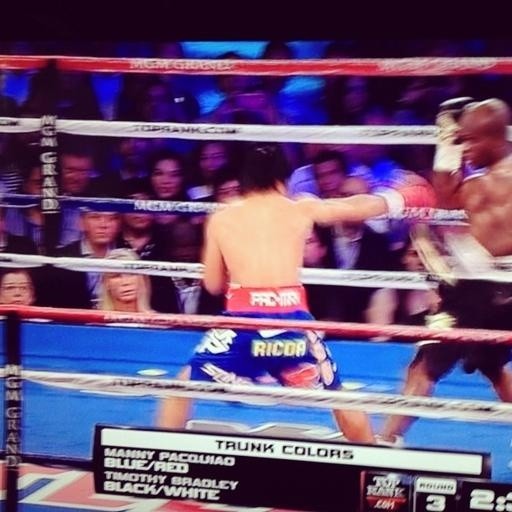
370,173 -> 439,222
432,96 -> 475,174
